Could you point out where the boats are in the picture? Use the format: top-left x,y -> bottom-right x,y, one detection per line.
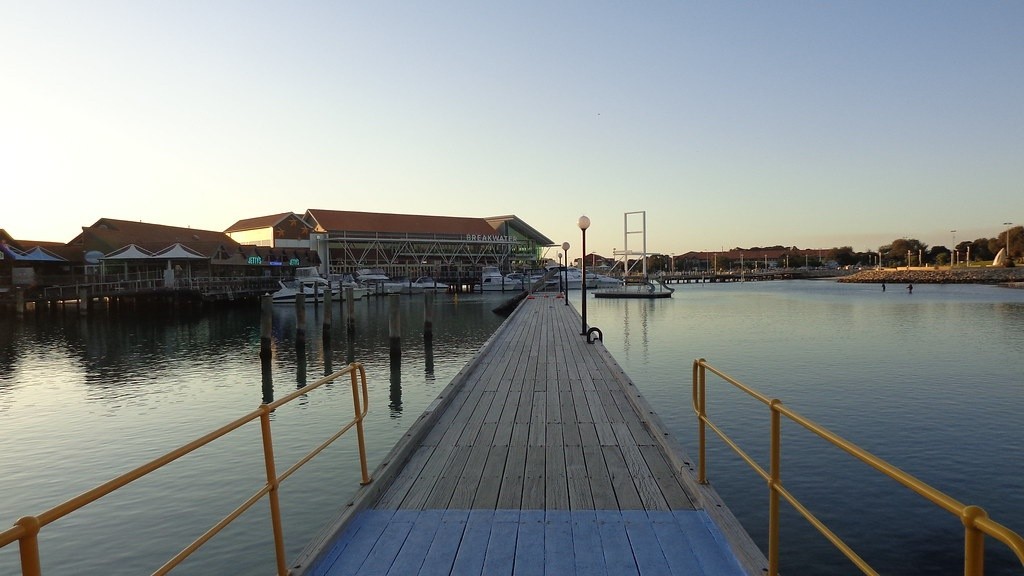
473,266 -> 517,291
401,275 -> 449,289
265,278 -> 339,304
356,274 -> 402,295
507,266 -> 624,291
319,273 -> 364,301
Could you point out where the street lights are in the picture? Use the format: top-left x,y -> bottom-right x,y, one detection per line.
558,253 -> 563,293
577,215 -> 591,335
562,241 -> 570,305
